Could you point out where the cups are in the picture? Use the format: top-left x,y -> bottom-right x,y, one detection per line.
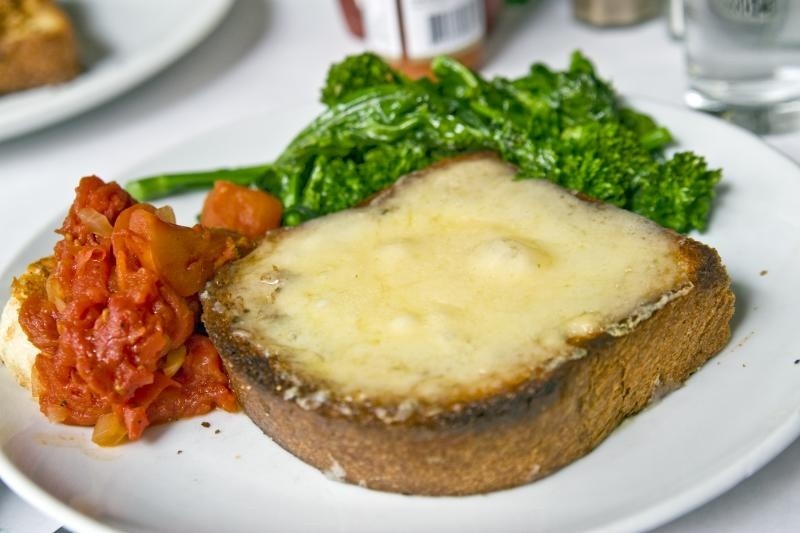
672,0 -> 800,135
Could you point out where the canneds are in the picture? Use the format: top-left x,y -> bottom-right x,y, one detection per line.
355,1 -> 498,82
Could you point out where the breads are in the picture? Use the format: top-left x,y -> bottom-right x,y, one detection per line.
0,0 -> 76,95
198,153 -> 736,496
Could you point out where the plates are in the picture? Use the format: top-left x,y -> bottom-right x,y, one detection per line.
1,0 -> 237,146
1,102 -> 800,533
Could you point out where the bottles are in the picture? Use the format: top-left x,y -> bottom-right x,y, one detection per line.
338,0 -> 491,84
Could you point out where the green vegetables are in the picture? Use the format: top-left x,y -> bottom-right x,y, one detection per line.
125,51 -> 723,235
